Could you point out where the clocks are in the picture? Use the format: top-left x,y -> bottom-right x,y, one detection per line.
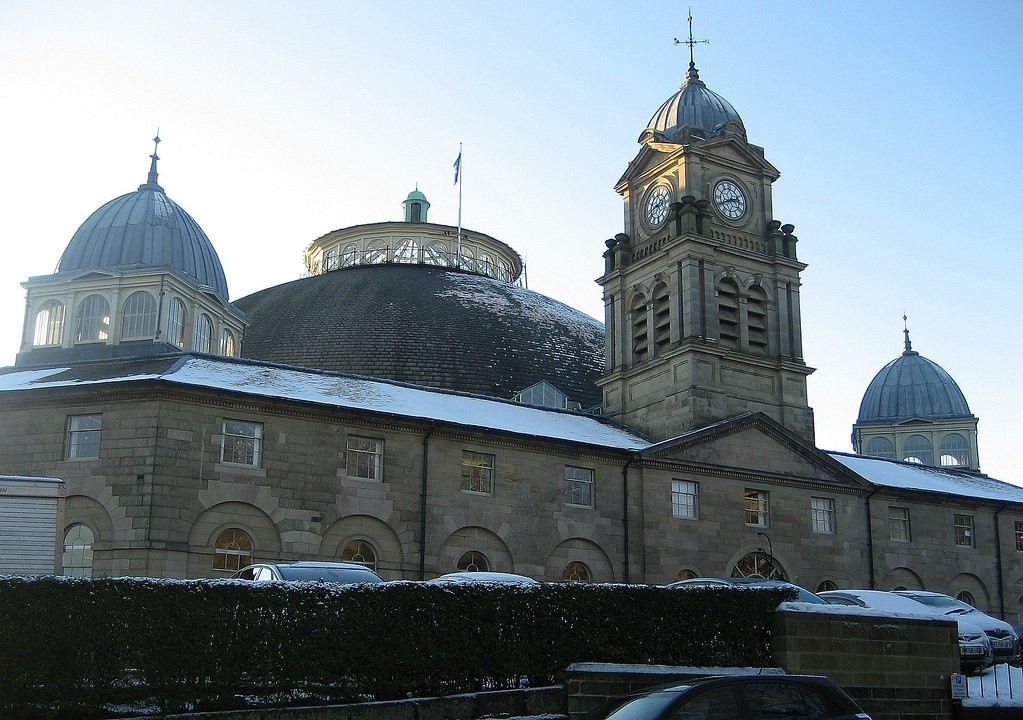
644,186 -> 671,229
713,180 -> 746,221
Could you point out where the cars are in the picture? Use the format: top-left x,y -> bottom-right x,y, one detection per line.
427,571 -> 539,584
228,560 -> 386,584
666,576 -> 833,613
890,589 -> 1021,667
567,672 -> 875,720
812,589 -> 992,675
